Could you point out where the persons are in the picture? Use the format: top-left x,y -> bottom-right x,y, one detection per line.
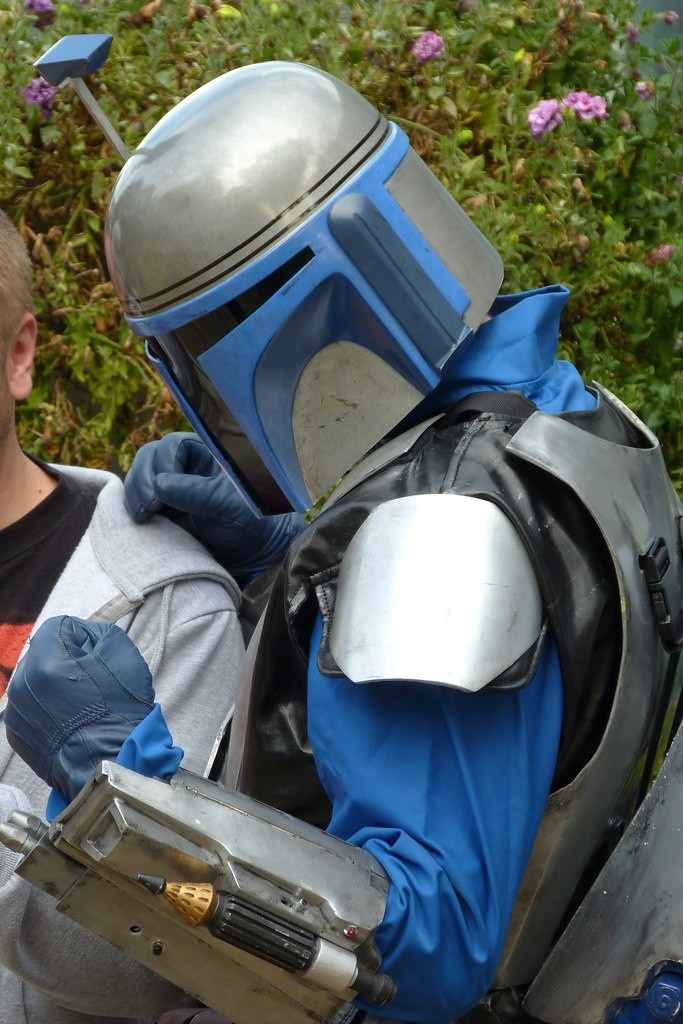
0,62 -> 683,1024
0,210 -> 246,1024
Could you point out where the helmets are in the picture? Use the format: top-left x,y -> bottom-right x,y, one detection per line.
99,58 -> 503,521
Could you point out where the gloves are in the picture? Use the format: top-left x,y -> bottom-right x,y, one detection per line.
119,429 -> 305,578
4,615 -> 154,805
